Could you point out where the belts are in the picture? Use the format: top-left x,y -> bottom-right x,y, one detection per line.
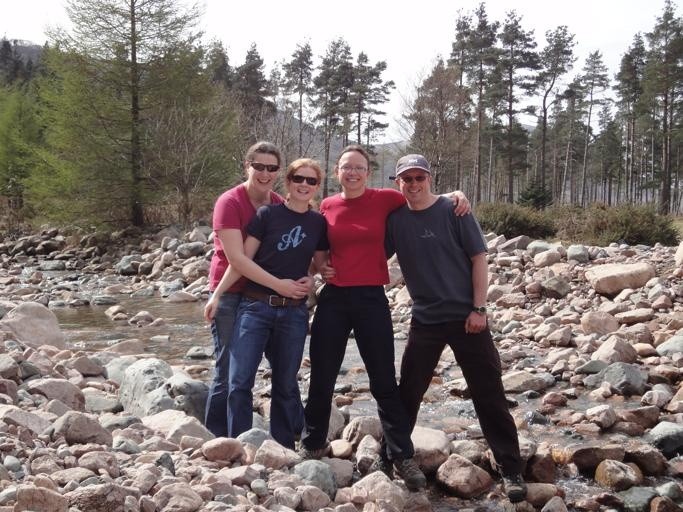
238,287 -> 313,307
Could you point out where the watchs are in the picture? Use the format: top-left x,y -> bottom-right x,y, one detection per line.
472,306 -> 486,315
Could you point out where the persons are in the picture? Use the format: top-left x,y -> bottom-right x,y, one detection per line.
206,140 -> 315,438
300,144 -> 472,493
320,154 -> 527,503
203,158 -> 330,450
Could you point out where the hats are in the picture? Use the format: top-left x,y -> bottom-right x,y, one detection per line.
395,153 -> 431,178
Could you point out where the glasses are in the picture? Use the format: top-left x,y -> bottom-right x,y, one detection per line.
339,165 -> 368,174
249,160 -> 281,172
402,175 -> 426,183
287,174 -> 320,185
